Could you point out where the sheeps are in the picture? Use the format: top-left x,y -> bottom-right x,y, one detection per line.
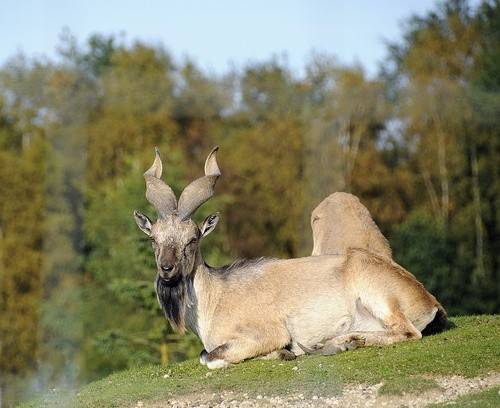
132,145 -> 446,369
